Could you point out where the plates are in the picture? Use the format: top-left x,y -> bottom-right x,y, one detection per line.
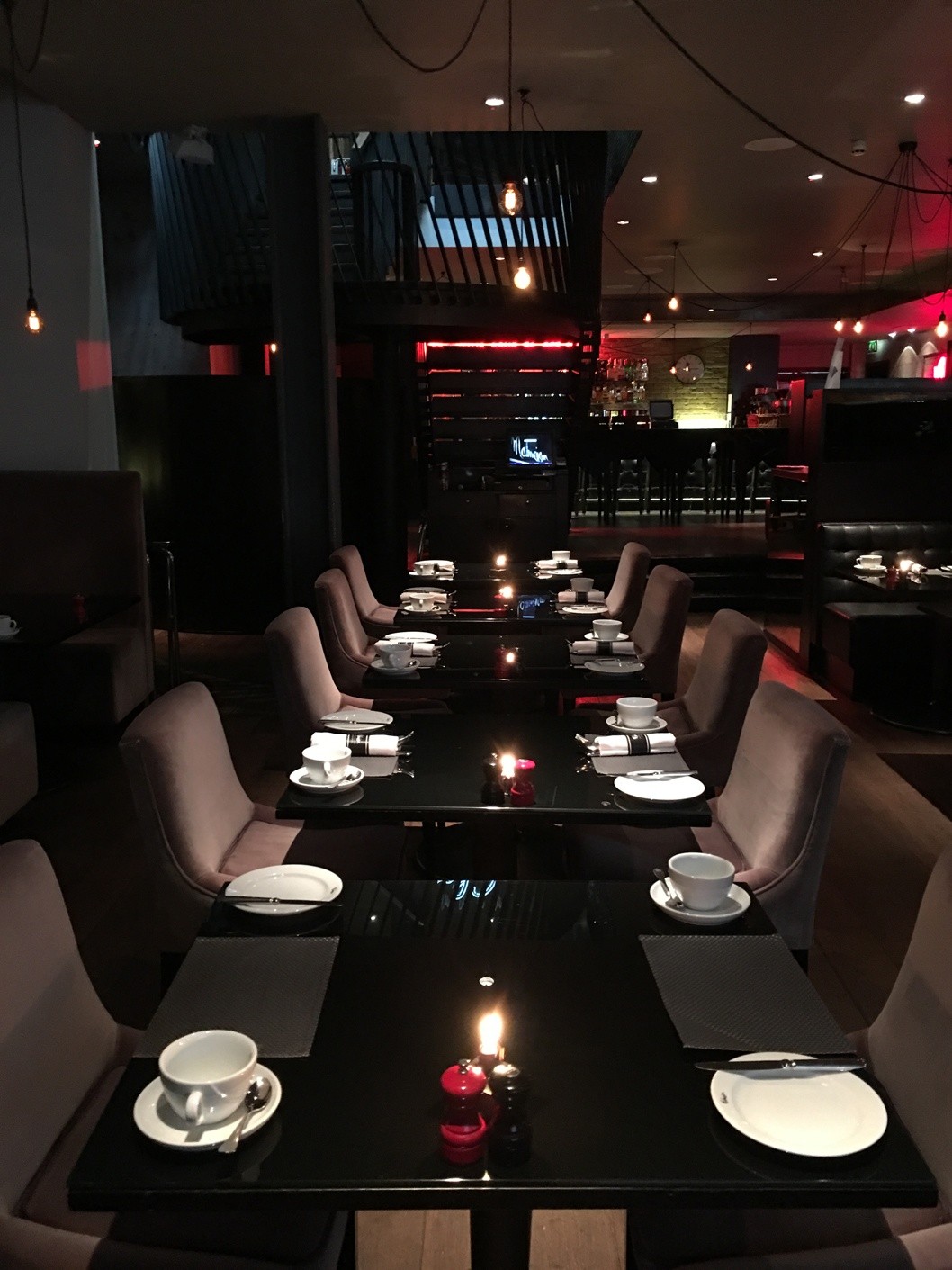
539,567 -> 750,926
320,711 -> 394,735
404,604 -> 442,612
133,1063 -> 282,1149
709,1050 -> 887,1157
370,658 -> 420,675
384,631 -> 437,642
225,864 -> 342,915
409,570 -> 440,577
289,764 -> 364,793
854,564 -> 886,574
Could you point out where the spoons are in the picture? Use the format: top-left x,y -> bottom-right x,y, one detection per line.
217,1077 -> 272,1153
324,771 -> 362,791
651,867 -> 683,910
403,660 -> 417,667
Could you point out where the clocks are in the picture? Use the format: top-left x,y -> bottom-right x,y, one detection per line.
674,353 -> 704,385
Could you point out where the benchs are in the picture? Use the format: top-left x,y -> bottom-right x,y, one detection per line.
0,468 -> 155,828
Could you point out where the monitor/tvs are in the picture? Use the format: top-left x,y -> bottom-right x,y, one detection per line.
649,398 -> 674,421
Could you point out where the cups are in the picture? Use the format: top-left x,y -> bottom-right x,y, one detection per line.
856,555 -> 883,568
615,696 -> 658,729
667,852 -> 736,911
302,744 -> 352,784
569,578 -> 594,592
380,644 -> 411,667
157,1029 -> 258,1126
593,619 -> 622,641
410,561 -> 435,610
552,550 -> 571,559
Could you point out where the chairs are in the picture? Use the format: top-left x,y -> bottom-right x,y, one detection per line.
691,679 -> 852,978
120,681 -> 305,950
603,541 -> 768,797
733,845 -> 951,1269
265,546 -> 397,743
0,840 -> 144,1270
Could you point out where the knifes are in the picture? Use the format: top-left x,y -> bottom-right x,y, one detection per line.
216,895 -> 343,908
317,719 -> 396,726
694,1057 -> 867,1072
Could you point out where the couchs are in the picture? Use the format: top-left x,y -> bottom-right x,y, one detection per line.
821,522 -> 952,701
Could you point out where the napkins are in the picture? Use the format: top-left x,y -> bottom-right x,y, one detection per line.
558,591 -> 605,603
594,733 -> 677,757
537,559 -> 578,569
572,640 -> 636,654
433,563 -> 454,571
400,593 -> 447,604
374,640 -> 435,656
311,732 -> 398,756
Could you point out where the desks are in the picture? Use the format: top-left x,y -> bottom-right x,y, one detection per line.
835,569 -> 952,735
67,880 -> 939,1270
393,590 -> 597,635
410,563 -> 578,590
276,713 -> 714,880
0,594 -> 148,725
566,428 -> 788,525
365,635 -> 650,713
